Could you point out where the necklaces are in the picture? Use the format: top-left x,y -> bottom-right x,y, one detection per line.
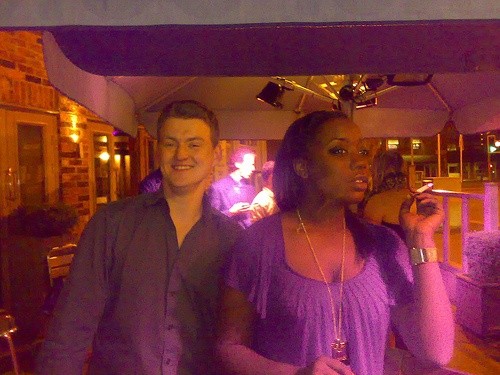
296,209 -> 351,366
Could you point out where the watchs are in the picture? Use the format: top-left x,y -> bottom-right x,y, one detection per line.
409,247 -> 438,266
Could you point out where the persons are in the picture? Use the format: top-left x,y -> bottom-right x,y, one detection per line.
34,100 -> 245,375
212,110 -> 455,375
208,147 -> 258,230
363,150 -> 417,352
250,161 -> 280,222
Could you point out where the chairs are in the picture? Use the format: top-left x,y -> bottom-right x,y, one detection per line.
47,244 -> 76,285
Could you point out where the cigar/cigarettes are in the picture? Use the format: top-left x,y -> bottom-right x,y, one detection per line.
407,183 -> 434,201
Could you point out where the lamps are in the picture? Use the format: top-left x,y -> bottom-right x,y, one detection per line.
256,80 -> 294,109
